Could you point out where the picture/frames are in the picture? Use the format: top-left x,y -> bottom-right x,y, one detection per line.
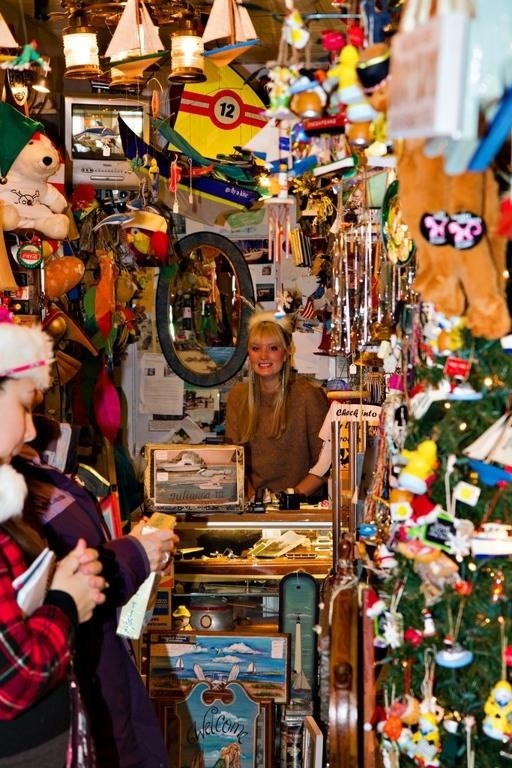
143,440 -> 247,514
142,630 -> 290,768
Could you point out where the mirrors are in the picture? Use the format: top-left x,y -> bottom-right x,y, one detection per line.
156,228 -> 261,389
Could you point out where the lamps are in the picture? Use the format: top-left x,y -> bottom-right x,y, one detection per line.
45,0 -> 212,89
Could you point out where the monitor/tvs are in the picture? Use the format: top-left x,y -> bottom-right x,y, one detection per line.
60,93 -> 151,191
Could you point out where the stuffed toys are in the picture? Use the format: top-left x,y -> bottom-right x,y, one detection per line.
0,133 -> 71,240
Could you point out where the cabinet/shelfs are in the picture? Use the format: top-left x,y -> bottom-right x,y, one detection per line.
126,504 -> 339,639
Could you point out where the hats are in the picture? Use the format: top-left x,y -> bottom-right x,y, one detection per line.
0,304 -> 52,392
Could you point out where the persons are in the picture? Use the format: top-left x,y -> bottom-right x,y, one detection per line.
226,321 -> 330,505
